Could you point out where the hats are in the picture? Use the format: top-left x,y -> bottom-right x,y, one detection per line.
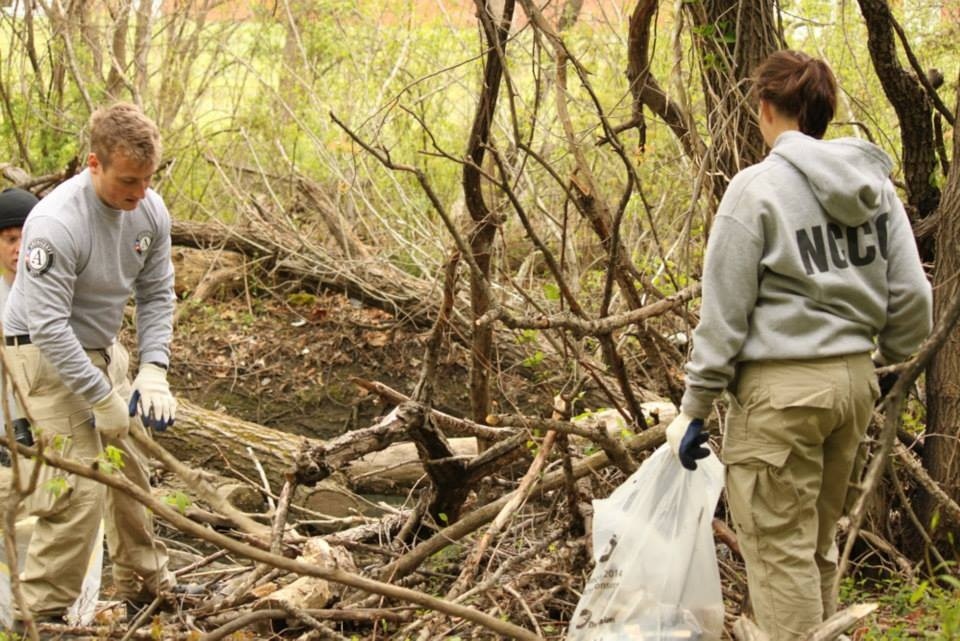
0,188 -> 38,231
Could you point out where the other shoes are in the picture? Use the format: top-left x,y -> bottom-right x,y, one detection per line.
10,616 -> 70,638
127,597 -> 196,625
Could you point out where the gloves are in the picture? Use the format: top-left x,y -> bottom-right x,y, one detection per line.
665,410 -> 711,471
871,348 -> 899,400
128,362 -> 177,433
4,417 -> 34,461
90,388 -> 130,440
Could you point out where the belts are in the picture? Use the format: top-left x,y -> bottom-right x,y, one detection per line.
5,334 -> 33,346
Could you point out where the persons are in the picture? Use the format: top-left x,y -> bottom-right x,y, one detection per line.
3,105 -> 177,641
679,50 -> 933,640
1,187 -> 38,511
